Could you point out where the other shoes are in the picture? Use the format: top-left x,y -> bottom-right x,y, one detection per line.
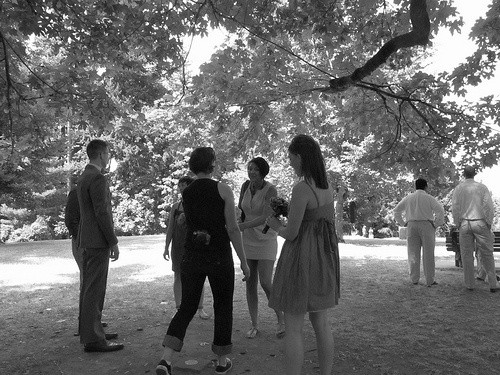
199,309 -> 207,319
246,327 -> 259,339
275,322 -> 286,337
431,281 -> 439,285
491,287 -> 499,293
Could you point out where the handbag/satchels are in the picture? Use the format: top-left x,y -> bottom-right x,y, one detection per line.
190,229 -> 215,248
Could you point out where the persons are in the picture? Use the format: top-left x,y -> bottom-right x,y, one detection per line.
65,139 -> 125,352
394,179 -> 445,286
162,176 -> 210,319
237,157 -> 286,337
266,134 -> 343,375
451,165 -> 500,293
155,148 -> 234,375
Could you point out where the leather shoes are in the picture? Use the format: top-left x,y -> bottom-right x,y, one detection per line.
84,341 -> 124,352
105,331 -> 117,339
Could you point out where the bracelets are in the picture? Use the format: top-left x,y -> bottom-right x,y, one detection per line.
277,225 -> 283,237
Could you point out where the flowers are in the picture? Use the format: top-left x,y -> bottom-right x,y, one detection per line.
176,213 -> 186,229
261,196 -> 288,234
235,206 -> 242,219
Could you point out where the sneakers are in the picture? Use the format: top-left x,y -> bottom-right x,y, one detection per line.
215,358 -> 232,374
156,360 -> 172,375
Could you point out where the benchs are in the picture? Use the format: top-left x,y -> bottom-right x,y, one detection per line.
445,232 -> 500,267
